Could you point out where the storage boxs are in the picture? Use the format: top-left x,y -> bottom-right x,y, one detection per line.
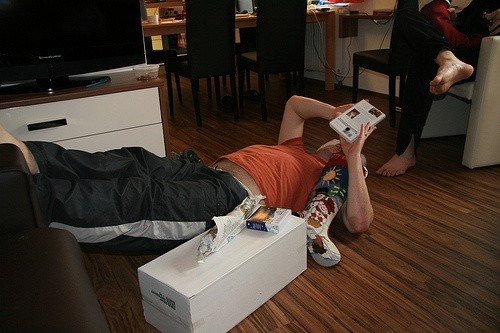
135,212 -> 307,333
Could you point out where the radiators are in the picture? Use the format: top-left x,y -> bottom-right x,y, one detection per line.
304,20 -> 342,76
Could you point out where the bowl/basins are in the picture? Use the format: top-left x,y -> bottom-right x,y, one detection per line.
133,64 -> 159,80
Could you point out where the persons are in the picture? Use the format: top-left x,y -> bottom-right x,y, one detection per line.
0,95 -> 376,255
376,0 -> 500,176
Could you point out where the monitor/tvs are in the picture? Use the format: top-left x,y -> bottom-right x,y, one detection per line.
0,0 -> 148,94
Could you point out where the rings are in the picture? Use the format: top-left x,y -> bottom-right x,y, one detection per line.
365,133 -> 367,136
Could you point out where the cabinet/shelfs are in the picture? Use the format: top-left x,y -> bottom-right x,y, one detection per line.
0,79 -> 167,160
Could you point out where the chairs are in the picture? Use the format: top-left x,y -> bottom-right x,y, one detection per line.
419,36 -> 500,169
352,0 -> 430,128
162,0 -> 239,127
232,0 -> 308,122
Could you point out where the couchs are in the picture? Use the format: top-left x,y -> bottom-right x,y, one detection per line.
0,142 -> 108,333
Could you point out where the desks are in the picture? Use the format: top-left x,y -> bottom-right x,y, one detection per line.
143,4 -> 335,91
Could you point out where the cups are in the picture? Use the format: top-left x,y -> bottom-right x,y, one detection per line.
147,15 -> 159,24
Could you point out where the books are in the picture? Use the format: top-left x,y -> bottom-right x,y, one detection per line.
329,99 -> 386,143
